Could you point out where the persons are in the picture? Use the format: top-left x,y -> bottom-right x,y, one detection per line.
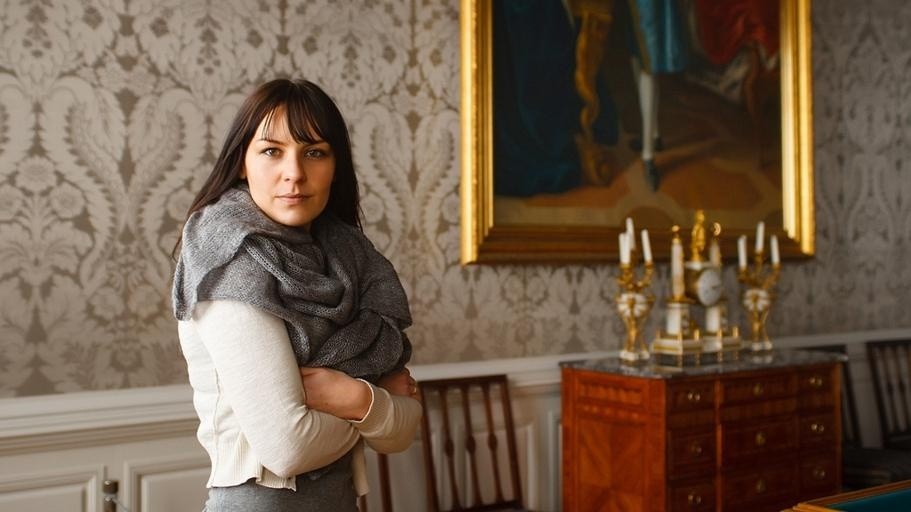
168,78 -> 426,512
622,1 -> 688,193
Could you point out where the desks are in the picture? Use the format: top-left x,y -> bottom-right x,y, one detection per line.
557,347 -> 852,511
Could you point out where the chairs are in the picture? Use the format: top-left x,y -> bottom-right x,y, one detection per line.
793,339 -> 910,491
375,372 -> 532,510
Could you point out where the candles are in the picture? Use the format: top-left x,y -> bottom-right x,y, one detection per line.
616,217 -> 654,268
736,220 -> 782,271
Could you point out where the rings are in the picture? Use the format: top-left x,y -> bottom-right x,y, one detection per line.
411,386 -> 419,395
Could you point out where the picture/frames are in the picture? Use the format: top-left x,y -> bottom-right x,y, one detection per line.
453,0 -> 820,267
